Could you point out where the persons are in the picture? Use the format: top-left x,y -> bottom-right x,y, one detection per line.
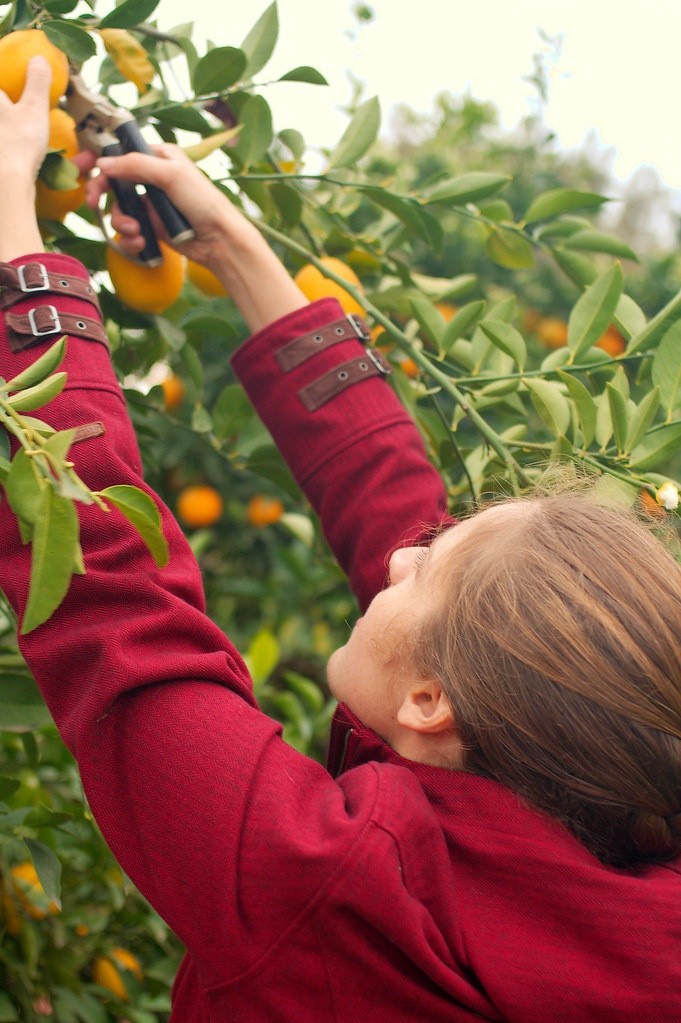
0,58 -> 681,1023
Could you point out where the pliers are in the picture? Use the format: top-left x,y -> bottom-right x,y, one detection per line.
64,55 -> 196,271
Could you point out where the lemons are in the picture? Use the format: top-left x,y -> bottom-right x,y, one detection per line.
0,24 -> 671,1002
